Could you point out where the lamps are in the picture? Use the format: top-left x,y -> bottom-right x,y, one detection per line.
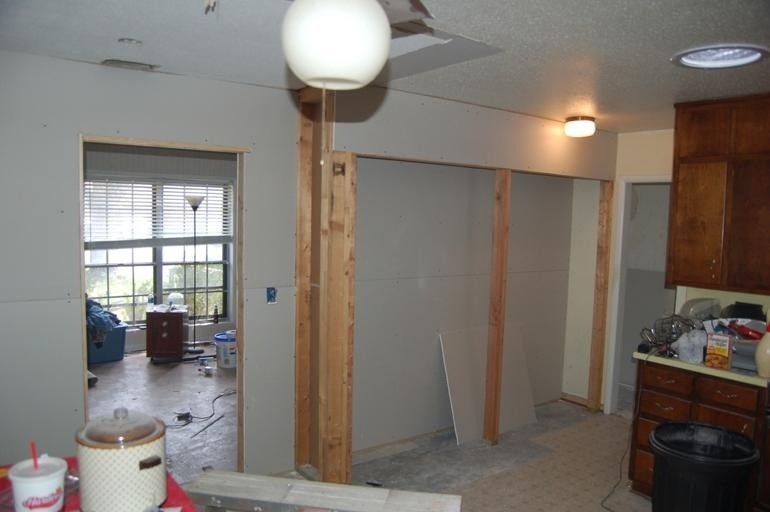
564,116 -> 596,138
184,195 -> 205,355
281,0 -> 393,92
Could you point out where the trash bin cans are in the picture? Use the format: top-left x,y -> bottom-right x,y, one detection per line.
650,421 -> 761,512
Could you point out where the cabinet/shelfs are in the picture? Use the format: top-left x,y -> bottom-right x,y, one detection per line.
146,305 -> 190,362
628,360 -> 770,501
663,93 -> 770,297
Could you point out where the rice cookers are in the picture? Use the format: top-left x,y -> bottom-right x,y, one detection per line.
73,405 -> 170,511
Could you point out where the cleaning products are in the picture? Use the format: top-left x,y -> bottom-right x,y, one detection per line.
213,304 -> 220,323
753,304 -> 770,379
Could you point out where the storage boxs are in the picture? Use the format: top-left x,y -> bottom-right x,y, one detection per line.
88,321 -> 128,365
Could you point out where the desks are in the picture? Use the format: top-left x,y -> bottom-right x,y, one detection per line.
0,455 -> 199,512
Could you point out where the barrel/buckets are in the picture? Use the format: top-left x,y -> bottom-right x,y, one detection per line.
214,332 -> 237,369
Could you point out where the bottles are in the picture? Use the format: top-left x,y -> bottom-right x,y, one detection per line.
194,357 -> 221,375
211,304 -> 220,324
146,293 -> 154,311
